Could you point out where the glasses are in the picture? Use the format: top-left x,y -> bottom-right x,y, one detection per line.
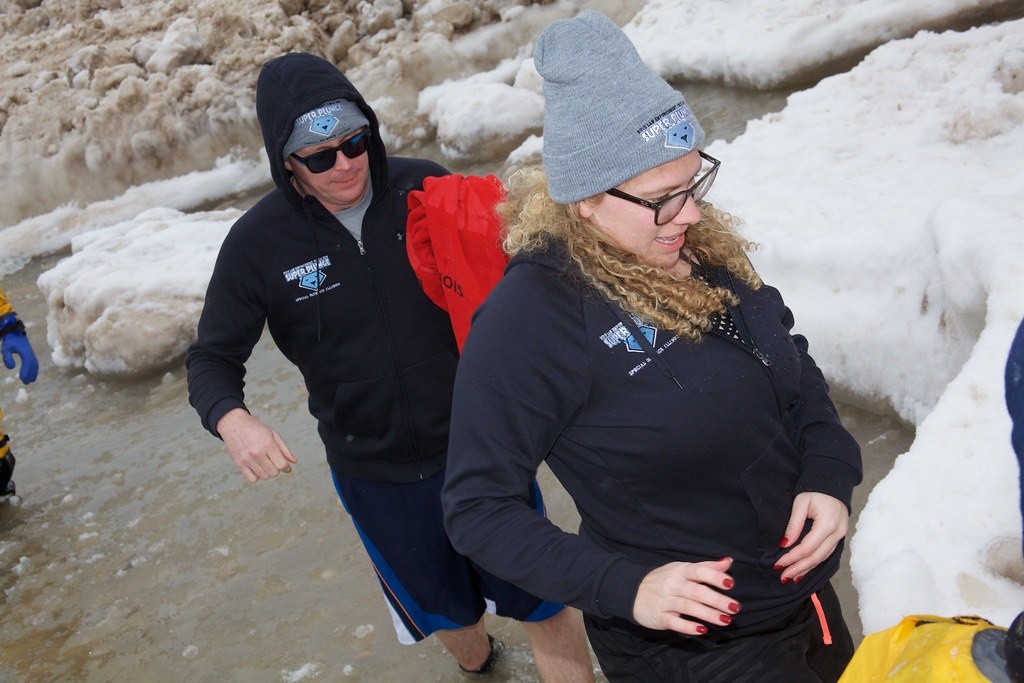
292,129 -> 369,174
604,150 -> 720,224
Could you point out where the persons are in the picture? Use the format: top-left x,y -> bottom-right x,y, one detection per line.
0,288 -> 39,510
186,54 -> 603,682
439,14 -> 867,683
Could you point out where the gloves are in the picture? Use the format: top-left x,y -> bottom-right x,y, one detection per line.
0,315 -> 39,384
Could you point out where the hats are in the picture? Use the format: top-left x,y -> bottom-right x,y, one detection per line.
281,98 -> 371,160
533,7 -> 706,207
